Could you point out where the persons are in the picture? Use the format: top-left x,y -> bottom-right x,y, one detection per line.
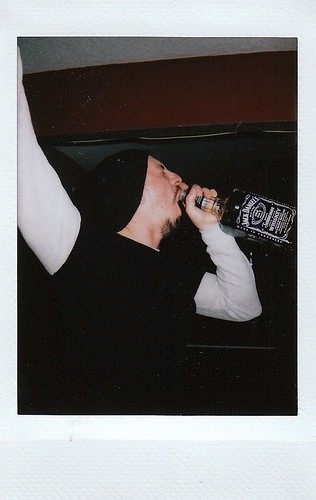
16,39 -> 263,416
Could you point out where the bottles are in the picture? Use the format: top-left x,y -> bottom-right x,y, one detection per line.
178,185 -> 296,254
